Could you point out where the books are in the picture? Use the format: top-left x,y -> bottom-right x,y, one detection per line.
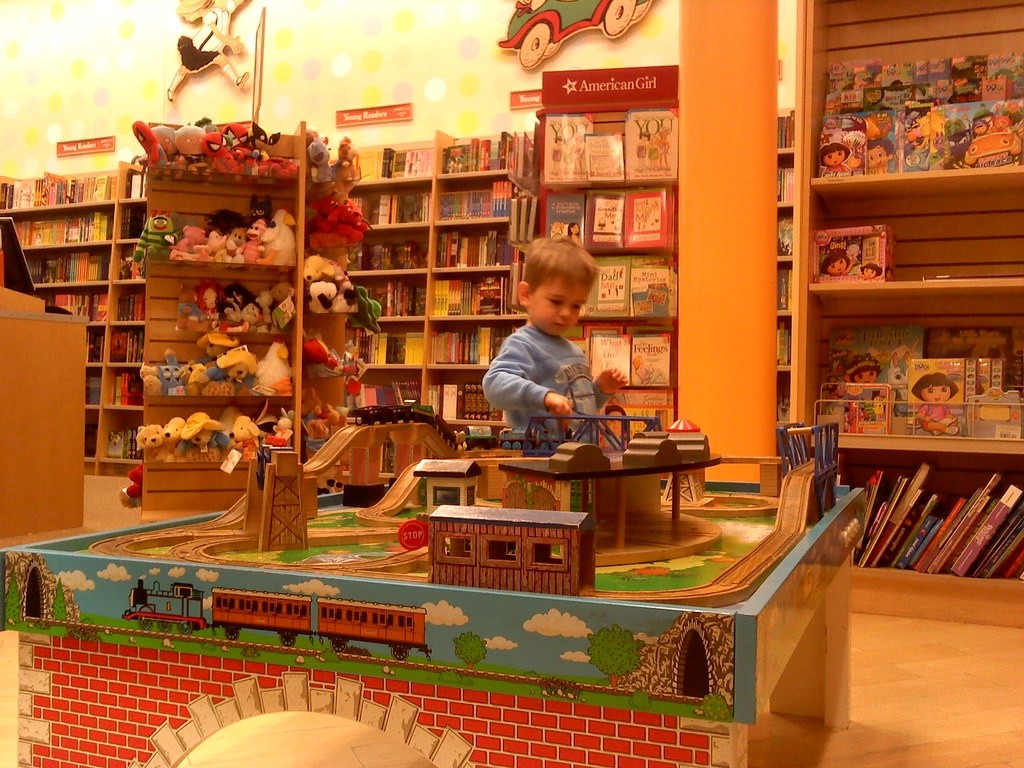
347,109 -> 678,449
854,464 -> 1024,580
0,172 -> 146,460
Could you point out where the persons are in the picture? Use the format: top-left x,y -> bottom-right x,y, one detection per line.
482,237 -> 629,442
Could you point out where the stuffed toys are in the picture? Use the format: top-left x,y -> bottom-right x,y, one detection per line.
121,119 -> 382,508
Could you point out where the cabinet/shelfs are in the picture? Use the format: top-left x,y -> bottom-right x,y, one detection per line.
796,0 -> 1024,627
0,108 -> 795,524
